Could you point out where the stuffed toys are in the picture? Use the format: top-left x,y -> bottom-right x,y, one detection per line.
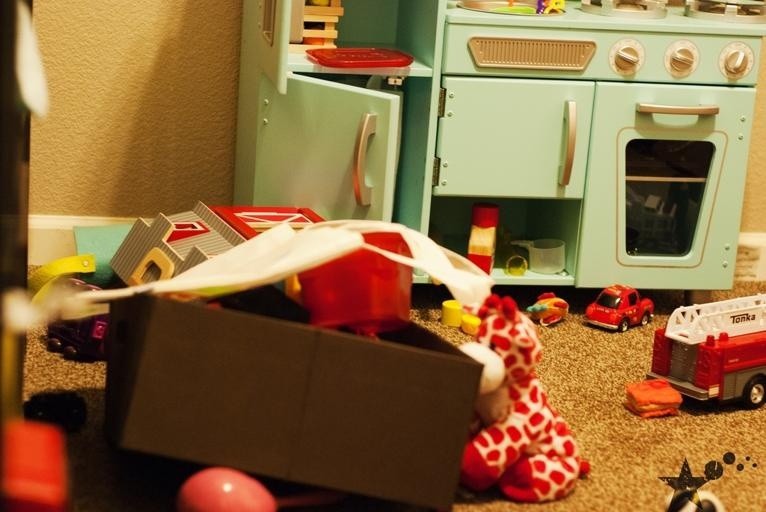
583,282 -> 766,409
458,292 -> 590,504
